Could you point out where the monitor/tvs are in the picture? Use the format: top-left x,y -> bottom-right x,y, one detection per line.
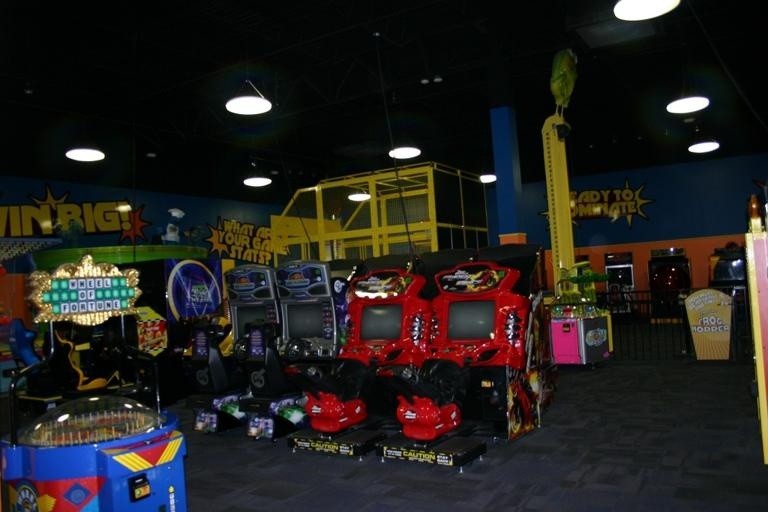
286,304 -> 324,338
360,304 -> 402,340
236,307 -> 269,338
648,258 -> 690,289
446,301 -> 497,340
606,265 -> 634,290
713,260 -> 744,280
